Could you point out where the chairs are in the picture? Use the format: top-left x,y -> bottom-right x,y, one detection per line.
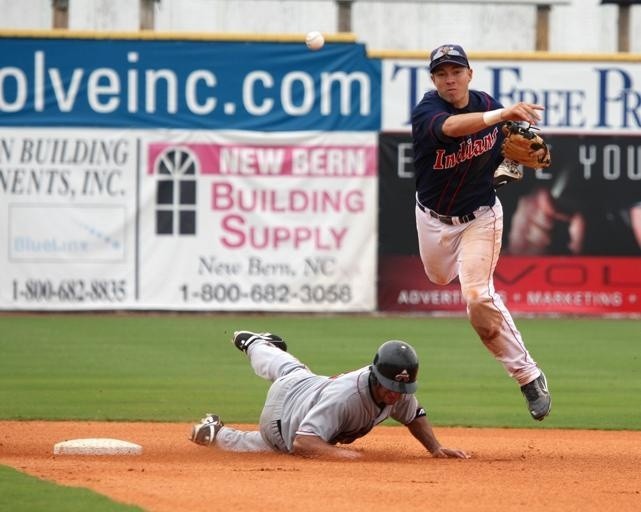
188,411 -> 225,449
231,330 -> 289,353
520,369 -> 553,422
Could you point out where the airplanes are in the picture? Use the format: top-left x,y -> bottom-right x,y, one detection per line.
501,121 -> 552,169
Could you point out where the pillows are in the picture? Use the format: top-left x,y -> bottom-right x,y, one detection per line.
369,338 -> 420,395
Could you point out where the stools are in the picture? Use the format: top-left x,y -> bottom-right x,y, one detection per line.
414,200 -> 489,226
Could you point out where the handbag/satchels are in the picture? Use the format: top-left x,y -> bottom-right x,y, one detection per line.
483,107 -> 505,127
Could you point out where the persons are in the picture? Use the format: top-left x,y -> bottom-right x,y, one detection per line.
509,133 -> 641,254
410,44 -> 555,422
189,329 -> 470,461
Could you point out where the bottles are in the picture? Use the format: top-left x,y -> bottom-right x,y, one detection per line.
429,43 -> 470,74
